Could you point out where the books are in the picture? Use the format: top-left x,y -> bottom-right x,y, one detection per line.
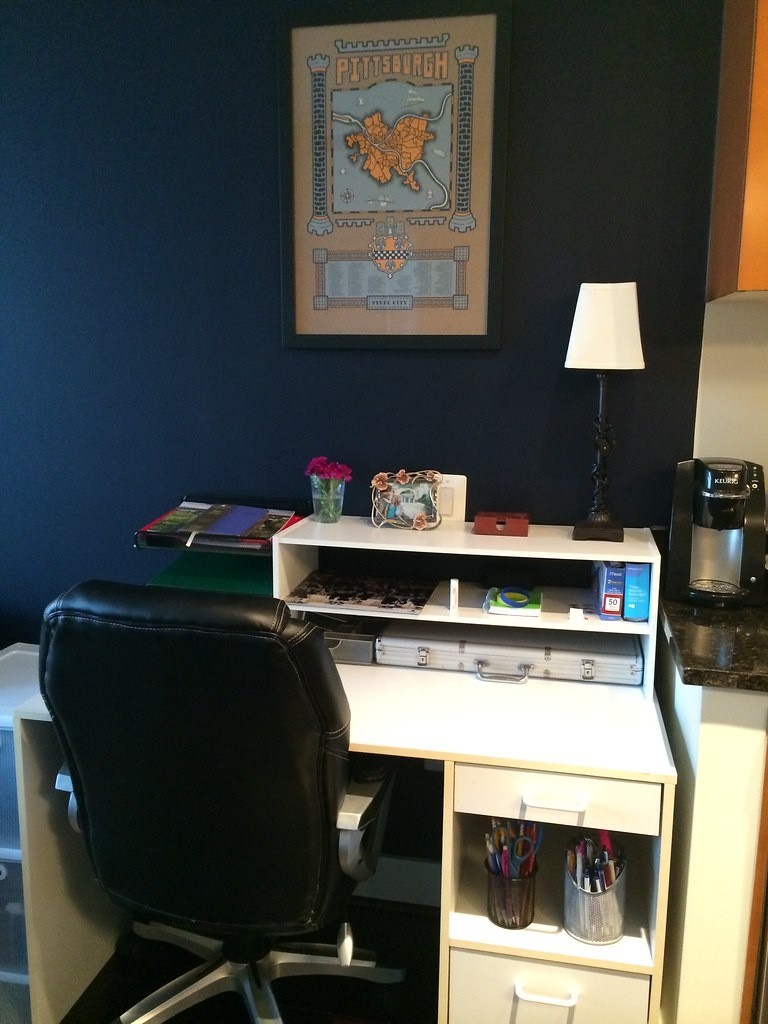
483,587 -> 543,617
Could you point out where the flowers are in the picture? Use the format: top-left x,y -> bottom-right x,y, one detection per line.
304,455 -> 352,523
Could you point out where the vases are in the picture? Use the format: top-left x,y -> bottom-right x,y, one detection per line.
309,475 -> 346,523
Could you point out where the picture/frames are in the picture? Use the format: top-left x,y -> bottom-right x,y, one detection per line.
273,0 -> 516,351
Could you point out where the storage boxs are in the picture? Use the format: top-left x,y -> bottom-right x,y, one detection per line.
0,639 -> 42,1024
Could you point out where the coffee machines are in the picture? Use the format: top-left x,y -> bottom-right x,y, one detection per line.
658,457 -> 768,692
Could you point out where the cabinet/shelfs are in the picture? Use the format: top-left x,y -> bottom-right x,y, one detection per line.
11,514 -> 681,1024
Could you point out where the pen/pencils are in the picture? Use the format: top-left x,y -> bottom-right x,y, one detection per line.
561,830 -> 627,941
483,816 -> 544,929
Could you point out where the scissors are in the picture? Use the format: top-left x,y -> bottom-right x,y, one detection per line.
491,824 -> 535,927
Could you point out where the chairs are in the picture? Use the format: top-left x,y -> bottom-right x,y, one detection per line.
37,575 -> 410,1024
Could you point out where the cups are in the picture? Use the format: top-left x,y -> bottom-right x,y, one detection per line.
563,839 -> 627,946
484,856 -> 538,929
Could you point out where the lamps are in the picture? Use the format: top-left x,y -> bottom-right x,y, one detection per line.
562,279 -> 646,541
